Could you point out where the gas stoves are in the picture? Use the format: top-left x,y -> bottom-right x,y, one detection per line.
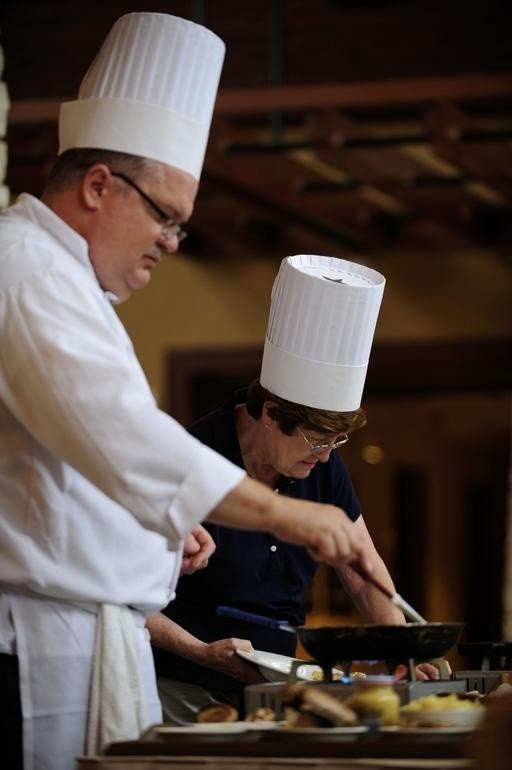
229,661 -> 467,723
452,660 -> 512,693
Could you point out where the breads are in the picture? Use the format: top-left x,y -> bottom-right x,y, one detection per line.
350,670 -> 367,681
312,666 -> 344,683
196,680 -> 400,729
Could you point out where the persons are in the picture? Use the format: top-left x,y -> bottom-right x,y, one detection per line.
0,148 -> 375,770
139,378 -> 454,720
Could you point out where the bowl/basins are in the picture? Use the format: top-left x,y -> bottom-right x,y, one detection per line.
399,710 -> 487,730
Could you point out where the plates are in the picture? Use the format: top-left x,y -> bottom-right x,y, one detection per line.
236,650 -> 344,683
152,726 -> 477,746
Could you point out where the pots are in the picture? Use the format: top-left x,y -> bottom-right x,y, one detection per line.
458,642 -> 512,658
215,604 -> 466,660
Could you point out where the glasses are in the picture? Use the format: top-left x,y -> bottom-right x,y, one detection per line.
292,424 -> 353,456
105,169 -> 191,245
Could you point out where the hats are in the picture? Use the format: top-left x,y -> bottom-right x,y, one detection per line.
54,10 -> 229,185
257,253 -> 389,417
